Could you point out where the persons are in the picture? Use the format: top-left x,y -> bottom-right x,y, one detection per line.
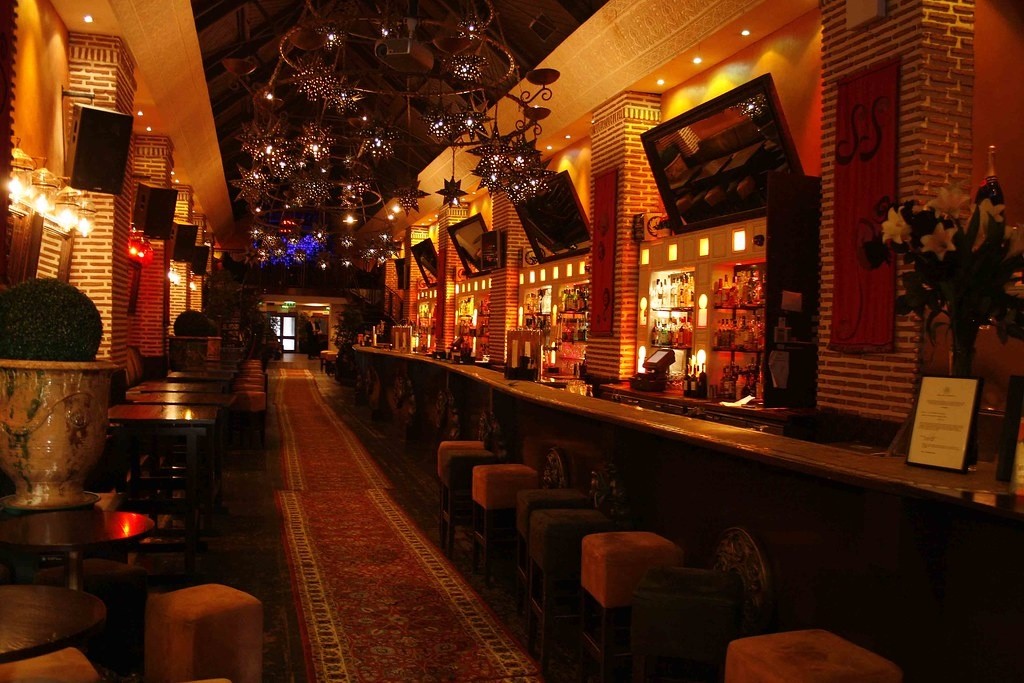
306,316 -> 323,360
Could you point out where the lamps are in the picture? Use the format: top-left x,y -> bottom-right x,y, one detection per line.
220,1 -> 563,267
5,136 -> 98,239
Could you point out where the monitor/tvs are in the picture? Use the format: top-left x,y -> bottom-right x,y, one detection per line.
480,229 -> 502,271
642,349 -> 676,369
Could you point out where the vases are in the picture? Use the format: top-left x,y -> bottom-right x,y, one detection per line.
938,308 -> 983,469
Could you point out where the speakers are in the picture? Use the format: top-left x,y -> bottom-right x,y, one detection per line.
132,182 -> 179,236
167,221 -> 198,261
190,243 -> 209,274
63,103 -> 134,195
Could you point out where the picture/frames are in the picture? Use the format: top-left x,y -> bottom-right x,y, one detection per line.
510,169 -> 599,262
446,211 -> 490,277
408,238 -> 440,289
639,71 -> 806,235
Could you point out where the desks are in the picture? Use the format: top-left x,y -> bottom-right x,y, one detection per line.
98,348 -> 238,554
0,509 -> 156,591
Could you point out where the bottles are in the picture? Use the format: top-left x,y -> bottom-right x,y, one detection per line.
521,283 -> 588,379
479,298 -> 490,361
562,380 -> 594,397
682,363 -> 708,398
709,264 -> 767,405
650,272 -> 694,347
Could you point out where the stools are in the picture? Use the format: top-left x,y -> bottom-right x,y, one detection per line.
513,485 -> 590,615
143,582 -> 263,683
630,564 -> 737,682
471,461 -> 539,584
319,349 -> 338,374
230,357 -> 266,449
525,507 -> 614,670
725,627 -> 904,683
436,438 -> 496,552
578,530 -> 684,682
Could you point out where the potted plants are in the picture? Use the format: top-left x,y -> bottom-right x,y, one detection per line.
0,277 -> 117,508
168,309 -> 210,370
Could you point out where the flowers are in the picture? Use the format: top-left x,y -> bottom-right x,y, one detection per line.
852,173 -> 1024,348
